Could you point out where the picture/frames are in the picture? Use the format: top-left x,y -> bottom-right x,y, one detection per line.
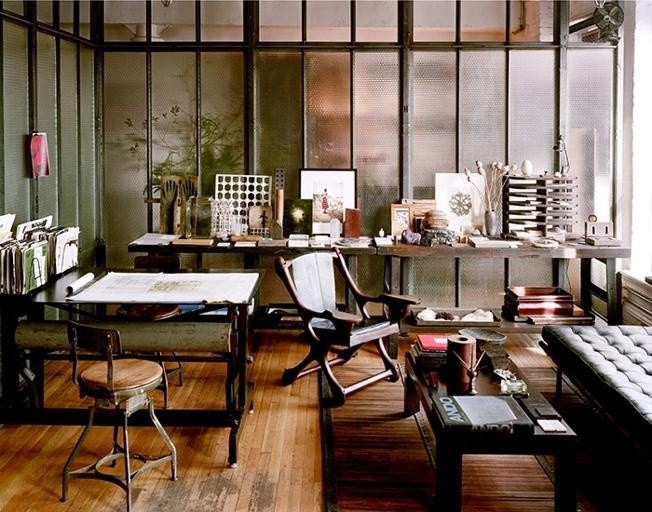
389,204 -> 412,239
296,167 -> 358,238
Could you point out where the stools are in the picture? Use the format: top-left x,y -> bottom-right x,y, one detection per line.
114,303 -> 179,391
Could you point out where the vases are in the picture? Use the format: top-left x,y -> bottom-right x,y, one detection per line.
485,210 -> 497,235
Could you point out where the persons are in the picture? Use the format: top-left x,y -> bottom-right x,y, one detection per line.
319,188 -> 330,215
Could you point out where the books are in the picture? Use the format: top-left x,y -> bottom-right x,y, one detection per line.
0,212 -> 81,297
431,390 -> 536,436
410,334 -> 458,364
466,236 -> 511,249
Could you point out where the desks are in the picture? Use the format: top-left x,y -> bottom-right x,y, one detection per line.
0,266 -> 268,468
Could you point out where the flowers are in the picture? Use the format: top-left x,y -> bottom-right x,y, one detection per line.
464,160 -> 517,212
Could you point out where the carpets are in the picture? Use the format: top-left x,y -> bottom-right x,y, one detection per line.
316,359 -> 586,510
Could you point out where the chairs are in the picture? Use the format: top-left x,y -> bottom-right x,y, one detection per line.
60,320 -> 179,512
266,247 -> 422,405
